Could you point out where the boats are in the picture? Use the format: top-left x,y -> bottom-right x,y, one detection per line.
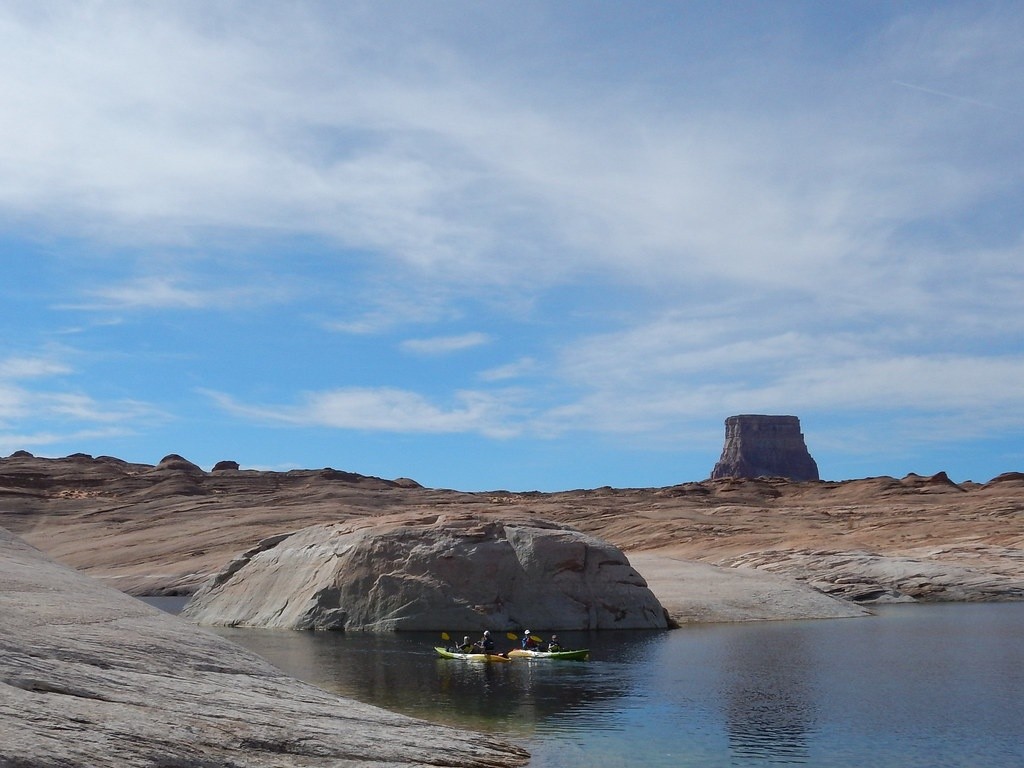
497,648 -> 590,659
434,646 -> 512,662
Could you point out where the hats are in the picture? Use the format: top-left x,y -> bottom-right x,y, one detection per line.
484,630 -> 490,636
525,630 -> 531,635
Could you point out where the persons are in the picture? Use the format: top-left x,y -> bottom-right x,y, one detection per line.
548,635 -> 561,653
447,636 -> 474,654
521,629 -> 544,652
474,630 -> 495,654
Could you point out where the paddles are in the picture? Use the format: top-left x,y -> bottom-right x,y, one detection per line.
441,632 -> 461,647
529,635 -> 552,645
507,632 -> 549,652
